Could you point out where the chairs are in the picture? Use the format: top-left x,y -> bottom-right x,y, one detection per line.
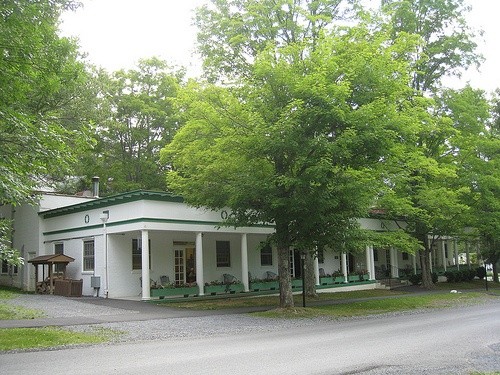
223,273 -> 238,292
160,274 -> 171,288
319,268 -> 326,278
138,276 -> 158,295
266,271 -> 278,280
